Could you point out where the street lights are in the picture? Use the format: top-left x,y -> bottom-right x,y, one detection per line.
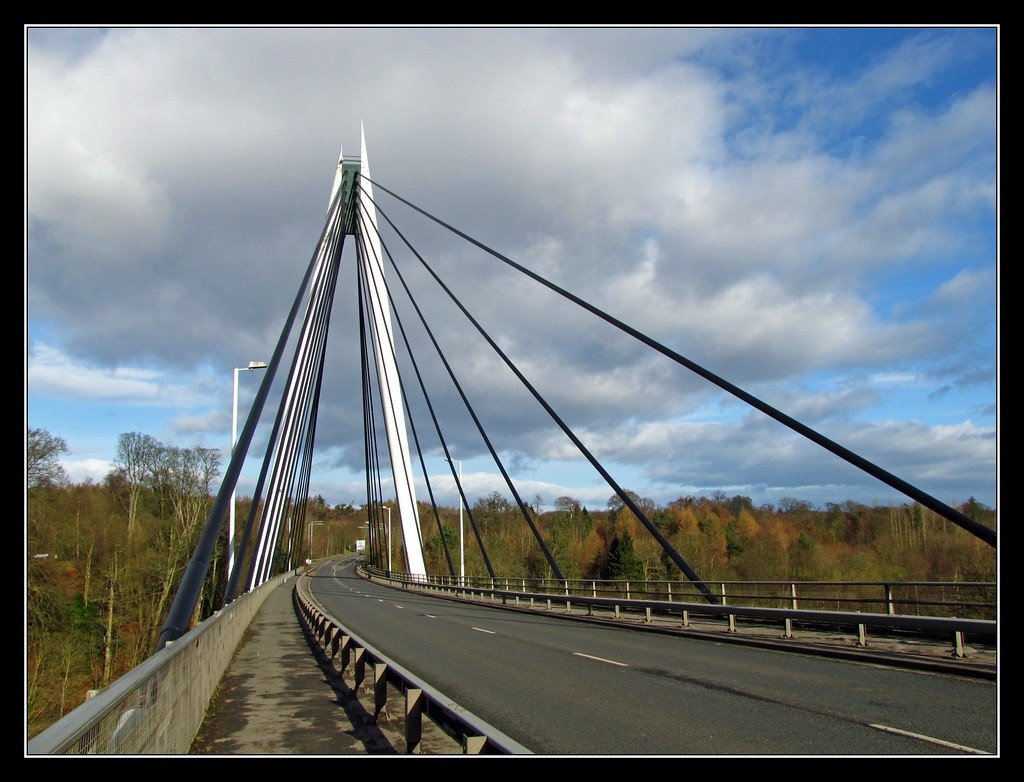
445,457 -> 465,587
226,361 -> 268,581
380,506 -> 392,574
